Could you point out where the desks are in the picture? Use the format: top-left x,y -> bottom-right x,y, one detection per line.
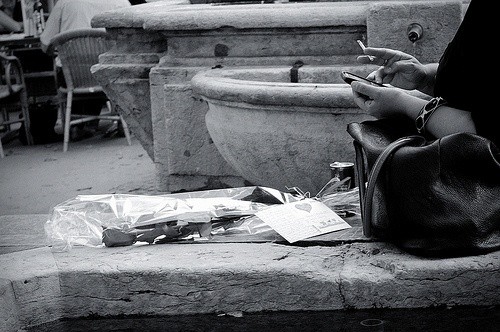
0,31 -> 59,109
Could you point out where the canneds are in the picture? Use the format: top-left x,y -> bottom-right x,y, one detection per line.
329,162 -> 356,217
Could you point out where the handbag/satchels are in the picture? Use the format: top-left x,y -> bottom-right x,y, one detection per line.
346,112 -> 499,259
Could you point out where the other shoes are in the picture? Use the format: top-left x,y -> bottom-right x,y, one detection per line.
70,128 -> 95,141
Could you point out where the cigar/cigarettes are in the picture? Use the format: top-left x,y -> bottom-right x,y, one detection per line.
356,40 -> 374,62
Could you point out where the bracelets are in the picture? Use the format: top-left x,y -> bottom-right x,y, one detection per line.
414,96 -> 448,135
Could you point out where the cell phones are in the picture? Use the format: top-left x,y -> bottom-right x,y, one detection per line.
340,71 -> 386,100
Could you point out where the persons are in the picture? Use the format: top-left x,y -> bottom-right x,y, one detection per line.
350,1 -> 499,140
0,0 -> 62,145
39,0 -> 133,139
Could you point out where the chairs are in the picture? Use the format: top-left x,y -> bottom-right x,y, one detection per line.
0,52 -> 35,159
49,27 -> 133,152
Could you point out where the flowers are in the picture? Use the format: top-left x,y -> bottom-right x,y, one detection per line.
100,201 -> 357,249
